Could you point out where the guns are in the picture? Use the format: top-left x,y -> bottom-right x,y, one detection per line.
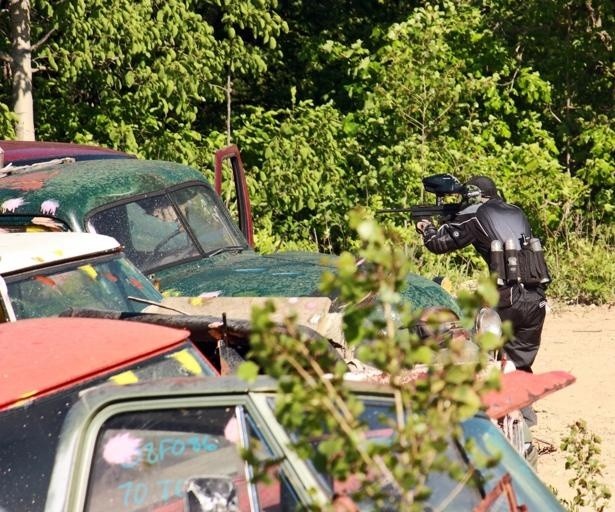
376,174 -> 469,233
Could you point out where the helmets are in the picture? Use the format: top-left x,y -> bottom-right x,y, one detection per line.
463,176 -> 498,206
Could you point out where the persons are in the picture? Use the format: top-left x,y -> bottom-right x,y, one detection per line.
413,174 -> 552,375
